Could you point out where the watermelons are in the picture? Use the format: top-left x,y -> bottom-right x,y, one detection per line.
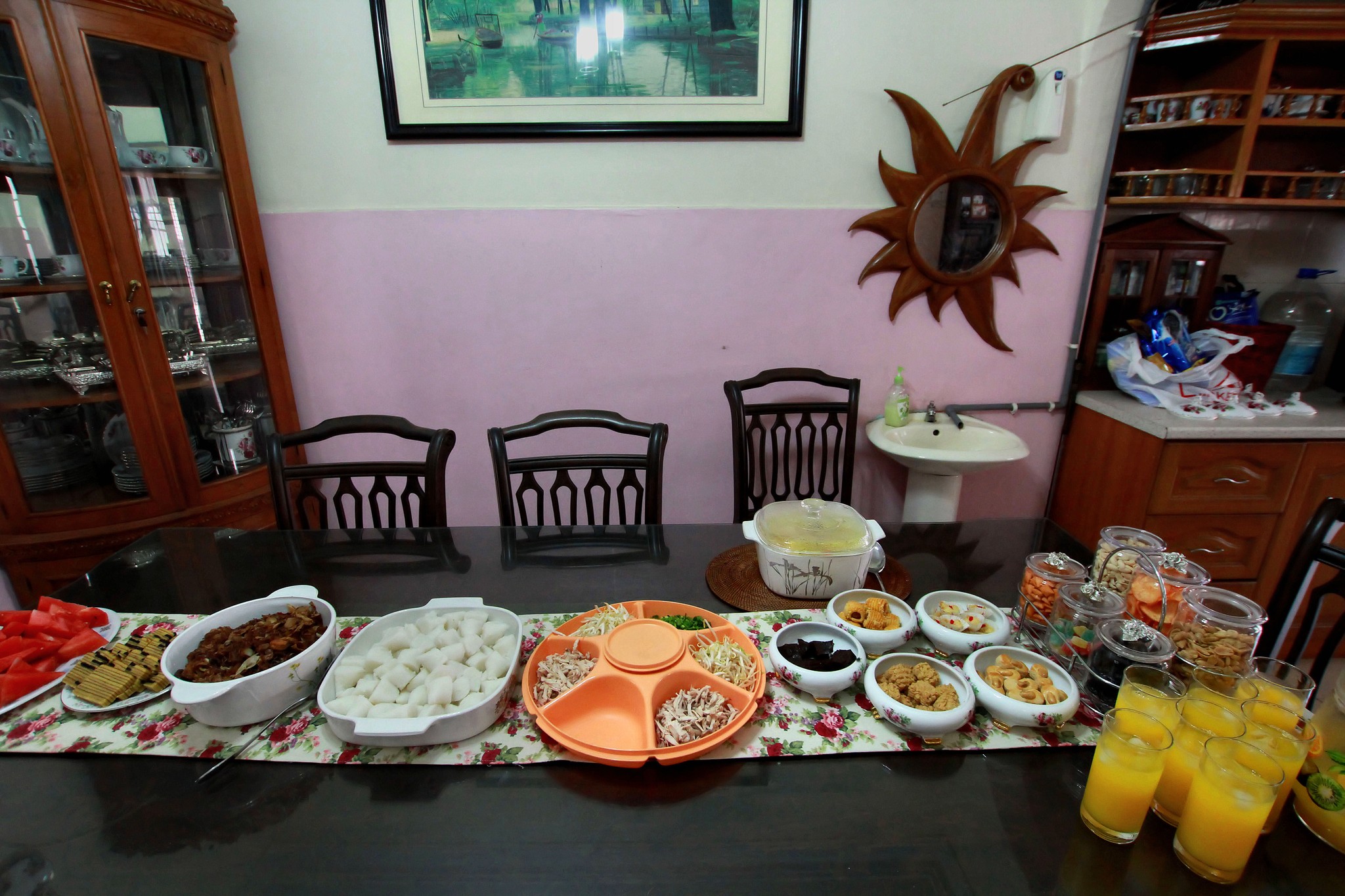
0,596 -> 110,709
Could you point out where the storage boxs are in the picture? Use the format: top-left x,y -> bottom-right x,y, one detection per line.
1199,319 -> 1297,393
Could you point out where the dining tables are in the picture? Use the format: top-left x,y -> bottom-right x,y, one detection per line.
0,518 -> 1345,895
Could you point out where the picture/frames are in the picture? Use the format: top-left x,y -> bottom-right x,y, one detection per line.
370,0 -> 807,139
972,203 -> 989,219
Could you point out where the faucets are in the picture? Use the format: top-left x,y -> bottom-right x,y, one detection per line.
924,401 -> 937,422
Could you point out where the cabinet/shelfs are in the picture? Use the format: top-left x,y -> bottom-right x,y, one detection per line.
1077,211 -> 1235,387
1106,0 -> 1345,209
0,0 -> 324,608
1046,405 -> 1345,658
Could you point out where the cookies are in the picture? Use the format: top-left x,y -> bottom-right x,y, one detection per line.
875,662 -> 960,712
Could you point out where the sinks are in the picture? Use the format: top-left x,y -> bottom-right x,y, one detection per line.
864,412 -> 1031,475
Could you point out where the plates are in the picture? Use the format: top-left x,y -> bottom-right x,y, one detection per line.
111,447 -> 148,495
141,254 -> 201,271
8,434 -> 87,495
189,435 -> 214,480
60,631 -> 184,713
0,607 -> 122,715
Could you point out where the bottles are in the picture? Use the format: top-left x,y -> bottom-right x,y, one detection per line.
1080,618 -> 1178,718
1258,268 -> 1332,401
1016,552 -> 1089,630
1167,585 -> 1269,689
1089,525 -> 1168,600
1123,551 -> 1211,638
1043,581 -> 1128,681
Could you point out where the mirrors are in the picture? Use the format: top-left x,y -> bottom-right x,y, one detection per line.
846,64 -> 1067,352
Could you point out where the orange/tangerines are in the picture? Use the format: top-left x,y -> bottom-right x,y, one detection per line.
1304,728 -> 1323,757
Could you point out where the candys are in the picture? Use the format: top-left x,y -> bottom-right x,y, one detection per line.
1048,618 -> 1097,663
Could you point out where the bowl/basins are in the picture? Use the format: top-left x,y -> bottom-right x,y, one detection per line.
914,590 -> 1010,657
204,248 -> 237,265
769,621 -> 867,699
160,585 -> 337,727
863,652 -> 977,739
741,498 -> 887,599
316,596 -> 522,747
962,645 -> 1081,728
826,588 -> 918,659
17,258 -> 54,276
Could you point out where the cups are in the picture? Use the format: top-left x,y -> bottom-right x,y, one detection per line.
1120,92 -> 1345,125
0,255 -> 29,278
51,254 -> 85,277
1079,655 -> 1345,886
129,146 -> 169,168
168,146 -> 208,168
212,418 -> 258,466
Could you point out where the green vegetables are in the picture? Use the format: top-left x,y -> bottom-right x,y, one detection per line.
1324,750 -> 1345,775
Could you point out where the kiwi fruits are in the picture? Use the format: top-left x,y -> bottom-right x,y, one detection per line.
1305,773 -> 1345,812
1296,759 -> 1319,787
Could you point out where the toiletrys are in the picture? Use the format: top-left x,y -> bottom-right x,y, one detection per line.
885,367 -> 910,427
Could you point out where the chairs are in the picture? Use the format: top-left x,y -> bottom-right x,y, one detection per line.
723,368 -> 861,525
486,410 -> 669,526
1253,497 -> 1345,712
266,414 -> 457,527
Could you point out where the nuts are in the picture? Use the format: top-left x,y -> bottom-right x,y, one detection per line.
1170,622 -> 1255,695
1091,538 -> 1154,599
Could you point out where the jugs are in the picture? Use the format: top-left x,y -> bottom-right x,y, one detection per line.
107,110 -> 132,167
0,93 -> 53,165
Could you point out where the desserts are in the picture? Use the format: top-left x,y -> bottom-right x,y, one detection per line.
928,600 -> 999,634
975,654 -> 1068,704
61,627 -> 183,707
836,597 -> 901,631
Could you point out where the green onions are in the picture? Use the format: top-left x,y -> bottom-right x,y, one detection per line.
648,614 -> 711,630
